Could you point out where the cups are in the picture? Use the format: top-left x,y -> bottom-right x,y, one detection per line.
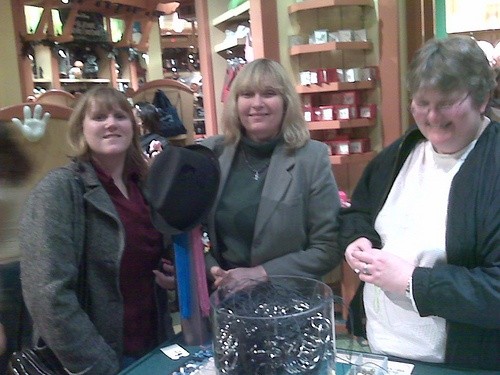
209,275 -> 337,375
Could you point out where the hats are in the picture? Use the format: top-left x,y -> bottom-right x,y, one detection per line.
144,143 -> 221,231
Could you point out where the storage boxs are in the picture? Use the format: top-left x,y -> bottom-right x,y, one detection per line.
302,91 -> 377,120
322,133 -> 370,156
298,64 -> 378,85
308,29 -> 368,45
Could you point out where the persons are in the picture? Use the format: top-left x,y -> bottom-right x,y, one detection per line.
200,58 -> 342,303
20,86 -> 177,375
132,102 -> 177,164
342,33 -> 500,371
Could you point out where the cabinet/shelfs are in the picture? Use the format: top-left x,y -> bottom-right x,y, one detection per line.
286,0 -> 378,165
12,0 -> 148,96
146,15 -> 207,137
212,0 -> 281,66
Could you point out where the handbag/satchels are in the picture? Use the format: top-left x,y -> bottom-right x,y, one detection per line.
7,346 -> 69,375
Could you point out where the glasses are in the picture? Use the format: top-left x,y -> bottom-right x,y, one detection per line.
409,90 -> 472,114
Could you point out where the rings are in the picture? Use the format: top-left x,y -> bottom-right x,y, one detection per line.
364,266 -> 369,274
228,290 -> 231,292
353,269 -> 359,273
405,283 -> 412,303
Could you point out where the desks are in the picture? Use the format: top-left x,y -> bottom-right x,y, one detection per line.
117,331 -> 500,375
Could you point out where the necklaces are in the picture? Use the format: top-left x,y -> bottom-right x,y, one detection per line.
241,147 -> 269,180
